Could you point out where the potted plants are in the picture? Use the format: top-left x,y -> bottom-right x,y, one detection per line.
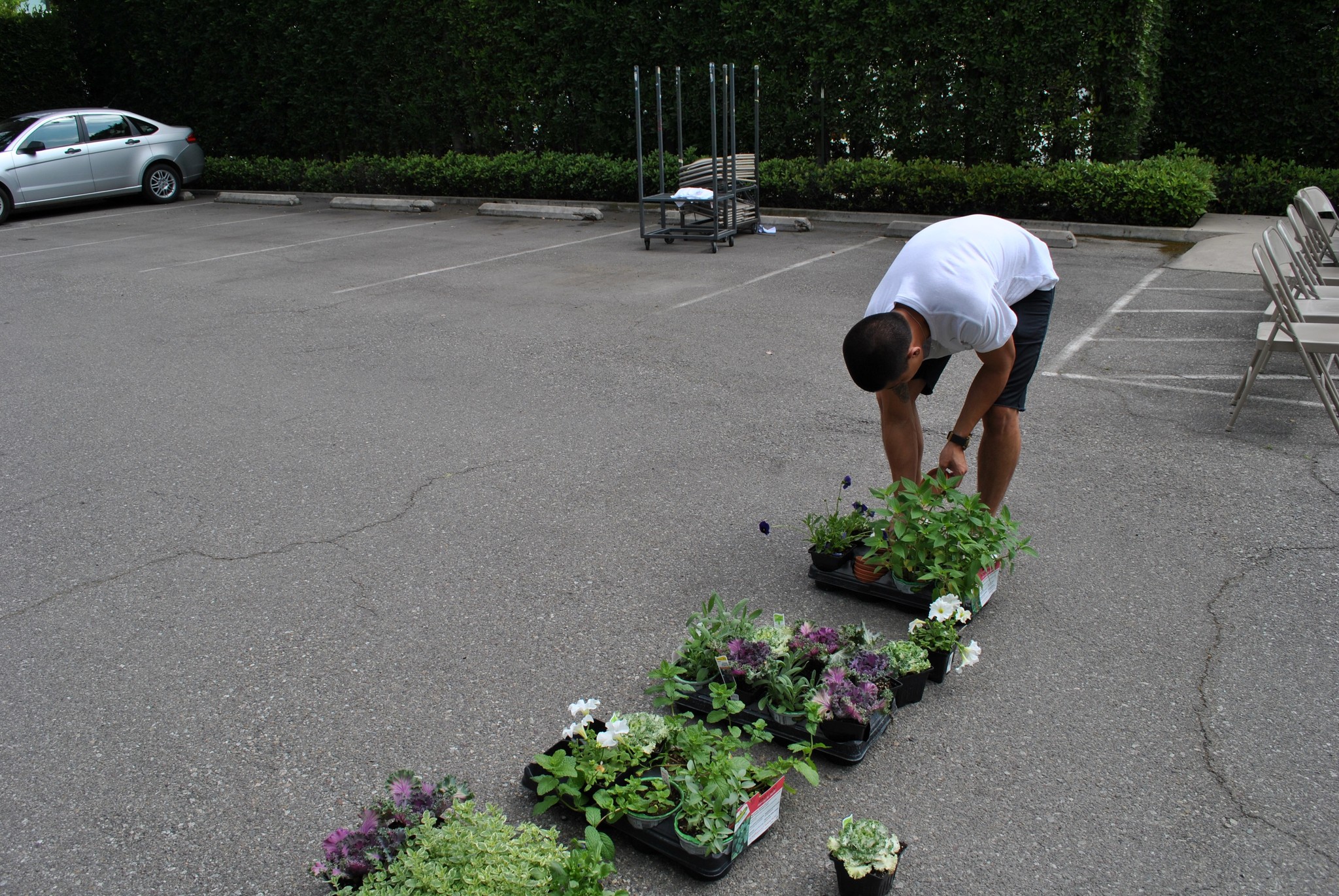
881,642 -> 934,706
311,597 -> 906,896
854,464 -> 1038,594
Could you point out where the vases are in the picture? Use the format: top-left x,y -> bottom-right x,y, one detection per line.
808,543 -> 845,570
723,672 -> 767,706
820,716 -> 870,743
922,636 -> 959,682
876,676 -> 903,708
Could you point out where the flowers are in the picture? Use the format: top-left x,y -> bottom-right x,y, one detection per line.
852,649 -> 895,683
805,665 -> 896,729
908,594 -> 982,675
757,476 -> 887,553
562,699 -> 636,771
709,638 -> 772,674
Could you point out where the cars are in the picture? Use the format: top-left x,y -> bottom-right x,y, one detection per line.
0,107 -> 206,226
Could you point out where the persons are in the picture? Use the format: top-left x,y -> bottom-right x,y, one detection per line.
842,214 -> 1060,539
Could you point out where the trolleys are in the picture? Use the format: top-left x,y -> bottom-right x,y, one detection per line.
631,58 -> 760,255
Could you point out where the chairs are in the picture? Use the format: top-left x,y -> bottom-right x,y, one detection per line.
1226,186 -> 1339,435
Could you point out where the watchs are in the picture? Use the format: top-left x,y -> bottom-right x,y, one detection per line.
946,431 -> 972,450
